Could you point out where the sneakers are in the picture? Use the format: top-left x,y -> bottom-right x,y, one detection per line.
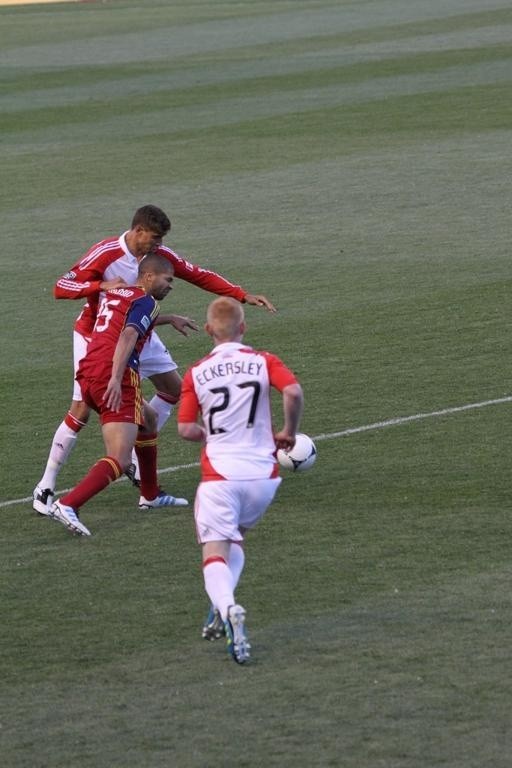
123,457 -> 142,489
202,601 -> 226,642
223,603 -> 252,665
32,484 -> 55,518
51,498 -> 93,536
138,493 -> 189,510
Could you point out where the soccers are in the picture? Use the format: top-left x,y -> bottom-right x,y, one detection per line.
277,434 -> 316,471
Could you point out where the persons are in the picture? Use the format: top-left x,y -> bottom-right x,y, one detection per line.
174,295 -> 305,668
47,254 -> 201,537
32,204 -> 278,518
